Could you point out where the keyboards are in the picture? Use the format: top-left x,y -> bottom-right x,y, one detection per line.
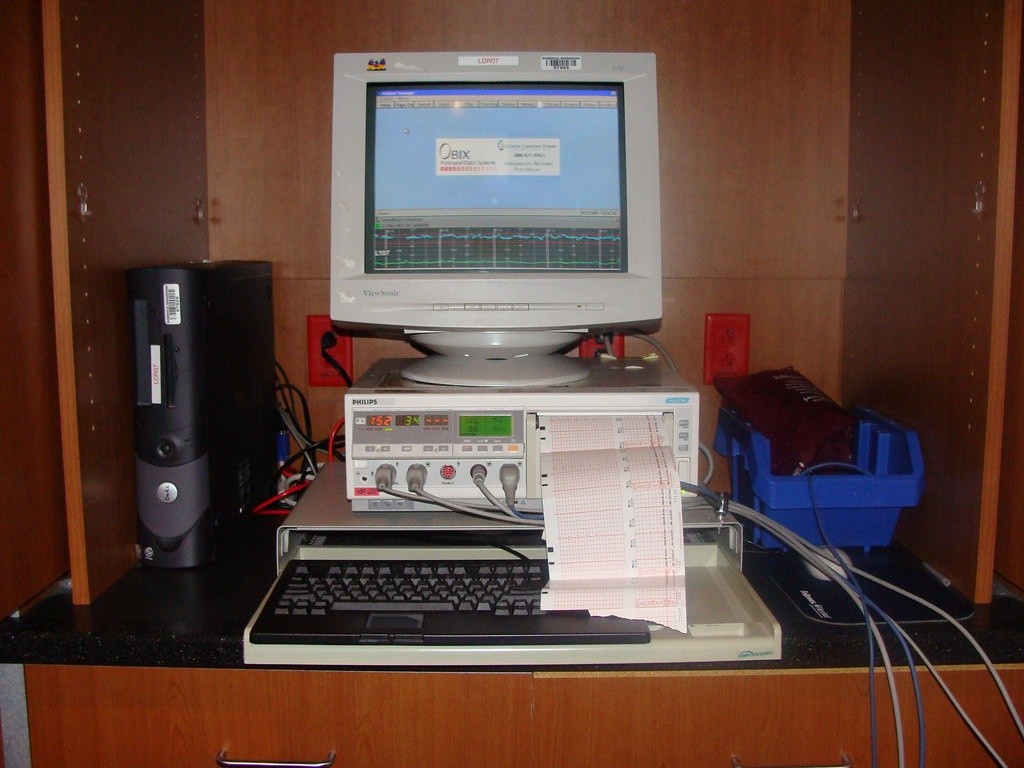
249,557 -> 650,645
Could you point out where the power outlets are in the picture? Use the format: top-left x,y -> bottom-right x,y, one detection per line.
703,313 -> 750,385
308,315 -> 353,387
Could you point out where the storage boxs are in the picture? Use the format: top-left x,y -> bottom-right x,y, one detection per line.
715,404 -> 928,552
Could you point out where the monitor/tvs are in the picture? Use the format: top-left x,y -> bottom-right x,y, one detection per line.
329,50 -> 663,385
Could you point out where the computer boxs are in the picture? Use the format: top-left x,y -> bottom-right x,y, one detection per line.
124,260 -> 277,568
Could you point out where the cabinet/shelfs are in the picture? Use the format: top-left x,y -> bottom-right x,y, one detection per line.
0,1 -> 1024,768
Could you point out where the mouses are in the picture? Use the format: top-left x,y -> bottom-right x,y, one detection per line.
804,544 -> 853,581
